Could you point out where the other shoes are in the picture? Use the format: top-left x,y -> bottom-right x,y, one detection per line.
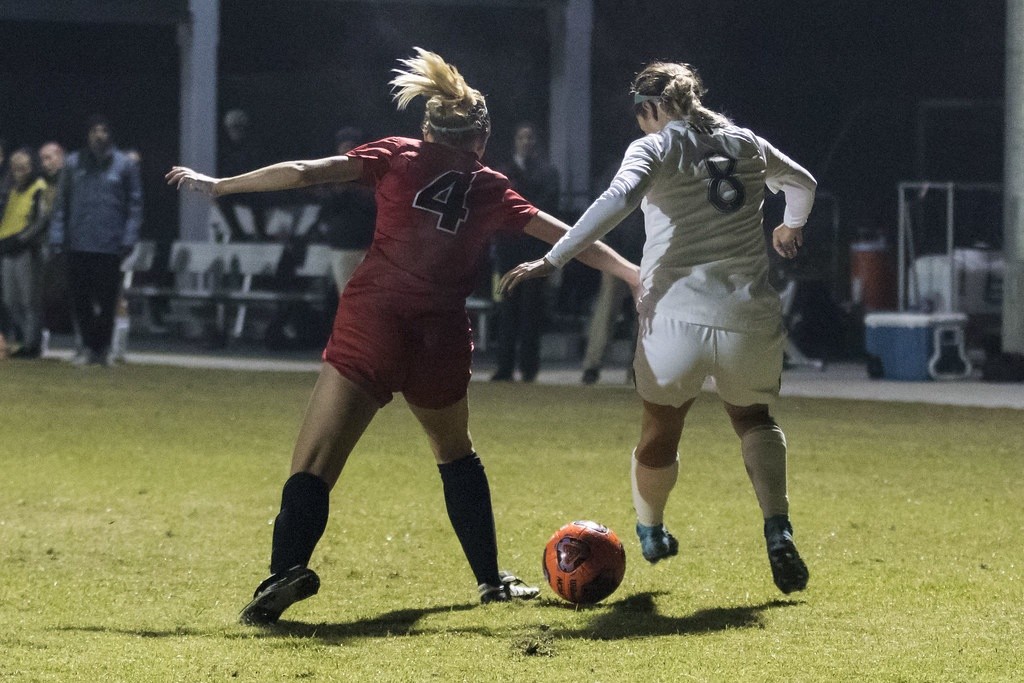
635,521 -> 679,563
764,515 -> 809,594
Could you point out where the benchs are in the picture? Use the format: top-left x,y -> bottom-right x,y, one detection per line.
125,242 -> 338,355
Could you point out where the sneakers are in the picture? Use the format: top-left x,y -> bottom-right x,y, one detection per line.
236,566 -> 320,627
476,571 -> 540,603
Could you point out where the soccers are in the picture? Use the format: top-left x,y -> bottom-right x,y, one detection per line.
541,518 -> 627,606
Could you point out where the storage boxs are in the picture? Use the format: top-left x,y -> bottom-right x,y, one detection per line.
865,311 -> 974,383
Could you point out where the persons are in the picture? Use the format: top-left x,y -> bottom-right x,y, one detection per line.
490,121 -> 559,381
317,127 -> 377,297
165,47 -> 641,624
496,61 -> 817,595
0,115 -> 142,364
576,159 -> 647,384
214,110 -> 280,241
